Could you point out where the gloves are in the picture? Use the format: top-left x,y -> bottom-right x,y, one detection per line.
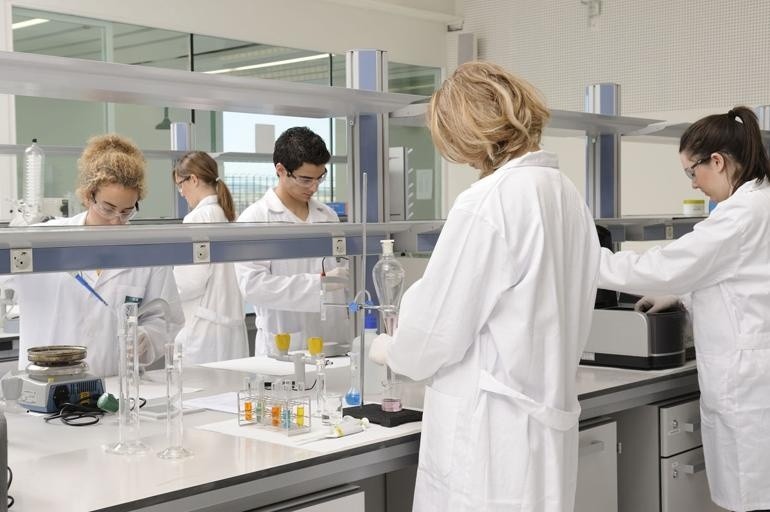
635,294 -> 678,314
326,267 -> 349,290
370,333 -> 391,366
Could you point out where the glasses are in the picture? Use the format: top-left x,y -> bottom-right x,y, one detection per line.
91,193 -> 138,221
685,157 -> 709,180
176,176 -> 190,191
289,168 -> 327,184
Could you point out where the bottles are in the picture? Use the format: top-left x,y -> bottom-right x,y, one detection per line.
113,301 -> 148,455
370,238 -> 406,386
314,352 -> 327,418
344,313 -> 386,404
318,271 -> 327,322
20,138 -> 46,226
242,374 -> 306,430
156,342 -> 195,461
683,200 -> 705,216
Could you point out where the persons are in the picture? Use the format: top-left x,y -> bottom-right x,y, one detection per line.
4,133 -> 185,383
598,105 -> 770,511
171,150 -> 251,368
233,125 -> 355,357
368,59 -> 603,510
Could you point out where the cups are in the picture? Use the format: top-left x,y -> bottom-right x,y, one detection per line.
379,379 -> 403,412
321,394 -> 344,428
